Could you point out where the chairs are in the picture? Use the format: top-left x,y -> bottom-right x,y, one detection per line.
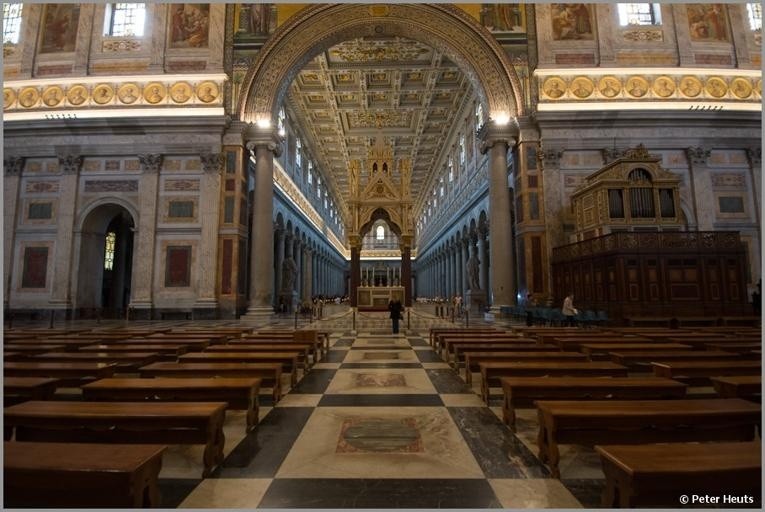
512,304 -> 610,327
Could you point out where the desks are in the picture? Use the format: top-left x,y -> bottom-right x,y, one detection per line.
2,323 -> 329,509
429,324 -> 763,509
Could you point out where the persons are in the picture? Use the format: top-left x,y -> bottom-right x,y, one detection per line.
524,294 -> 539,327
387,294 -> 405,334
546,79 -> 746,97
561,292 -> 578,327
454,292 -> 464,318
3,88 -> 215,107
667,318 -> 681,330
173,8 -> 209,47
312,294 -> 349,305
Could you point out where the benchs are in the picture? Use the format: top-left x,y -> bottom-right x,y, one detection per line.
160,311 -> 193,320
